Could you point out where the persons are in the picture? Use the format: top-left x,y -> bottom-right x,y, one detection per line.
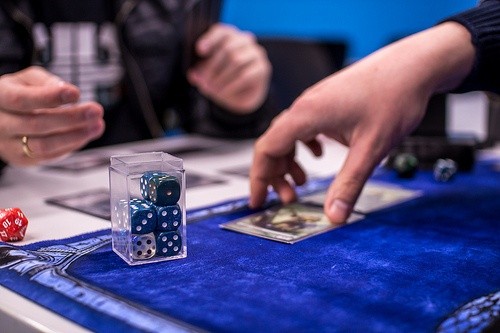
248,0 -> 500,227
0,0 -> 283,174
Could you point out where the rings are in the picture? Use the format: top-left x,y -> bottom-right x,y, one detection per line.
22,136 -> 38,159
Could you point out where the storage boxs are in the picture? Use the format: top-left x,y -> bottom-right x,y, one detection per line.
107,151 -> 187,265
